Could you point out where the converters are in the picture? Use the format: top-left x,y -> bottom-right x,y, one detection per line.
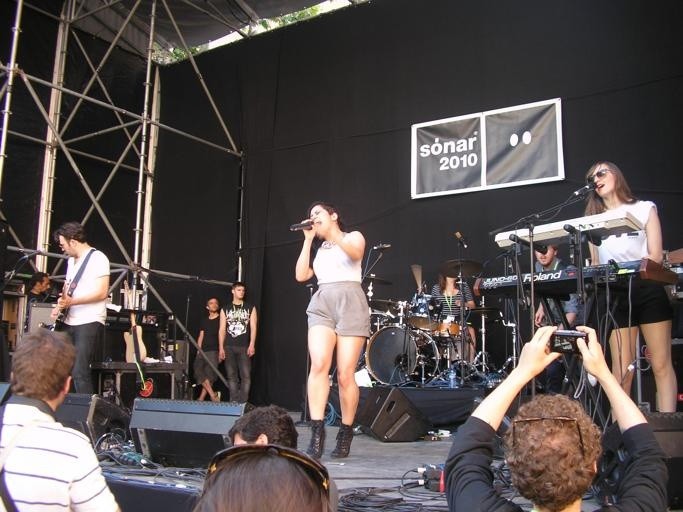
428,479 -> 439,491
425,468 -> 441,479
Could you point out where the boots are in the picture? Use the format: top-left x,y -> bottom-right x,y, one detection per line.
331,422 -> 354,458
307,420 -> 325,459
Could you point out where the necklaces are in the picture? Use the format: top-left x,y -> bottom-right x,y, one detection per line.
320,232 -> 346,249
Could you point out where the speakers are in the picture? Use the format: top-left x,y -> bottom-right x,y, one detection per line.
157,340 -> 189,400
53,392 -> 131,456
360,385 -> 433,442
129,397 -> 256,475
591,411 -> 682,505
97,369 -> 174,416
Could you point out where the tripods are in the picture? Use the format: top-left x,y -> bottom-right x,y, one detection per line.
389,278 -> 544,387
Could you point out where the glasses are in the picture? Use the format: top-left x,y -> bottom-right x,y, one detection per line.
588,169 -> 611,184
206,444 -> 330,501
512,416 -> 586,458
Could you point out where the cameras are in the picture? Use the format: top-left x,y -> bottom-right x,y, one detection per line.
551,330 -> 590,355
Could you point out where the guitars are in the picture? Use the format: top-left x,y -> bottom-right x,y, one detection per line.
123,281 -> 147,362
52,279 -> 69,331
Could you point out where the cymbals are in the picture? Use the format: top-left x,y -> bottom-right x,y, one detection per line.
466,305 -> 501,315
362,276 -> 393,286
367,299 -> 412,316
437,259 -> 483,276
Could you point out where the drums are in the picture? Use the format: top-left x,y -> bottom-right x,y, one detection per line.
432,319 -> 461,338
409,295 -> 443,330
364,324 -> 438,384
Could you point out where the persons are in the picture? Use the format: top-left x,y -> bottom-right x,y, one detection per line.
581,160 -> 678,424
216,282 -> 259,403
187,445 -> 339,511
0,329 -> 121,512
50,219 -> 110,394
22,271 -> 50,332
532,244 -> 582,385
293,200 -> 371,460
190,295 -> 222,401
430,258 -> 476,364
228,403 -> 298,451
441,325 -> 670,512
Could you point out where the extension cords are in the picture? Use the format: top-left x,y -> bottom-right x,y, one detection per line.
439,470 -> 444,494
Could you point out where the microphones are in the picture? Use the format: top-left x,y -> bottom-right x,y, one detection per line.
454,232 -> 468,249
289,221 -> 314,232
25,248 -> 45,258
373,243 -> 391,250
573,183 -> 597,198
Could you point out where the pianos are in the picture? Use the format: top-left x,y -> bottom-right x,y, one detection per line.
472,259 -> 681,297
